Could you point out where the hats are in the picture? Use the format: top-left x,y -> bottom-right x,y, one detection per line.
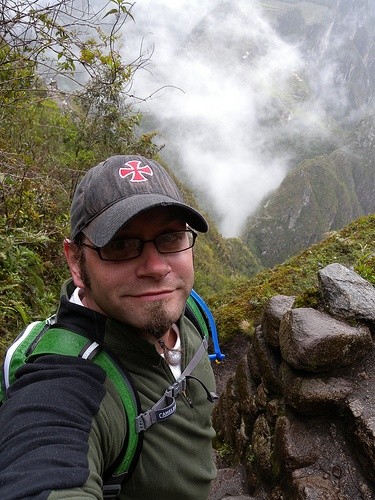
70,155 -> 207,248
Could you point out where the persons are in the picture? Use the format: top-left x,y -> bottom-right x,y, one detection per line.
0,152 -> 225,500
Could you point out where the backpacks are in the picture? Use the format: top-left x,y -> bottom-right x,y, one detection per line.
1,296 -> 209,500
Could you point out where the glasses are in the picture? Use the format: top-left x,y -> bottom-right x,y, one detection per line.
80,227 -> 197,261
